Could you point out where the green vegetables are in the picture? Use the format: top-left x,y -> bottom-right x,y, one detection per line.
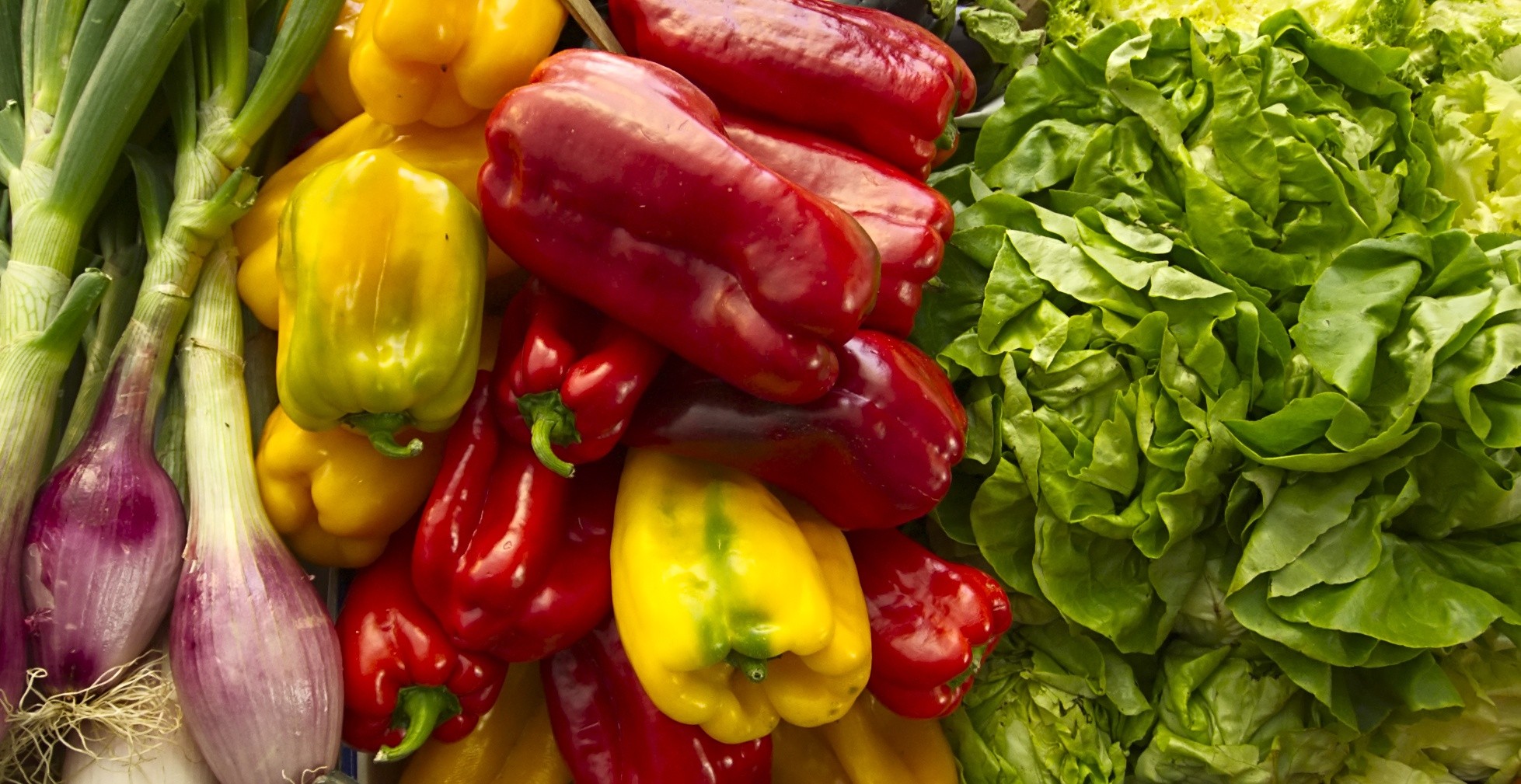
912,2 -> 1521,783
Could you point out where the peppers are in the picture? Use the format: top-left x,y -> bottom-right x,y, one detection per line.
231,0 -> 1014,784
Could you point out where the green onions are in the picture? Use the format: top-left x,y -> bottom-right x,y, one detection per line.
0,0 -> 361,784
